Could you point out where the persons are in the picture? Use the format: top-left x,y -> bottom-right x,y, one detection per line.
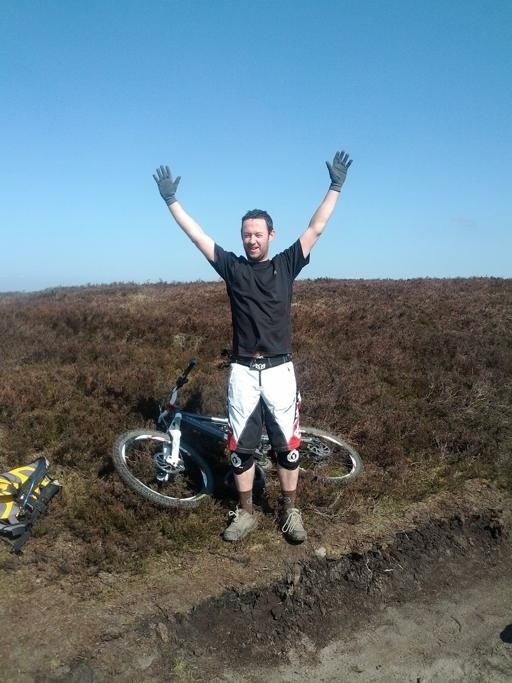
151,149 -> 358,544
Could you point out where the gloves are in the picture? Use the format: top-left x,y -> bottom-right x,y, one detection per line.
149,163 -> 185,212
323,147 -> 354,196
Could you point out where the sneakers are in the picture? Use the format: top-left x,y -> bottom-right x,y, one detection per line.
277,501 -> 308,546
223,502 -> 259,545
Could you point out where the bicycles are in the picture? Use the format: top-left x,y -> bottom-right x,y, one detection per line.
110,358 -> 364,510
